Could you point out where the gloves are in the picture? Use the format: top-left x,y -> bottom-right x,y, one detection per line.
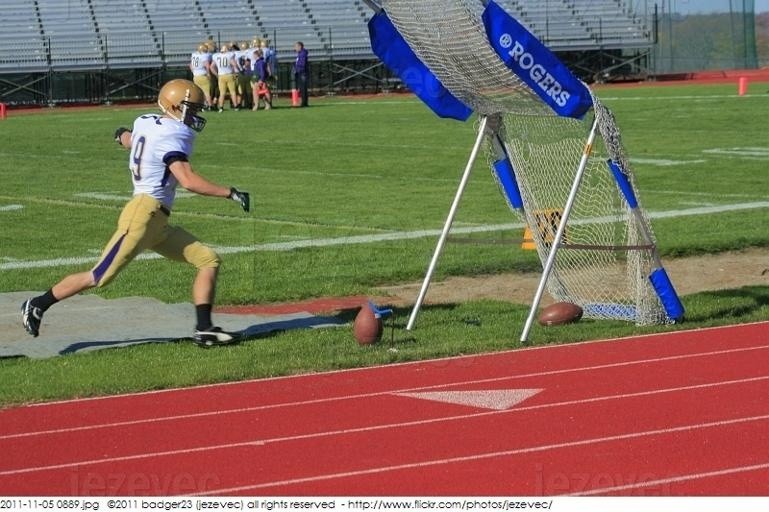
114,127 -> 130,145
226,188 -> 249,213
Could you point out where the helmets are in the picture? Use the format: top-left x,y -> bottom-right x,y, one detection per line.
198,38 -> 269,52
158,79 -> 207,132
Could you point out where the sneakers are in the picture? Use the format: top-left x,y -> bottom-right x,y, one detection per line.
22,297 -> 42,338
193,325 -> 242,348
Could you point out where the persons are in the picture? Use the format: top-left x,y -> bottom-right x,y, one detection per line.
292,41 -> 309,107
21,79 -> 250,349
190,37 -> 274,114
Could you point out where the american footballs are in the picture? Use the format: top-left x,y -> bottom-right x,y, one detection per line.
539,302 -> 582,325
355,304 -> 383,345
